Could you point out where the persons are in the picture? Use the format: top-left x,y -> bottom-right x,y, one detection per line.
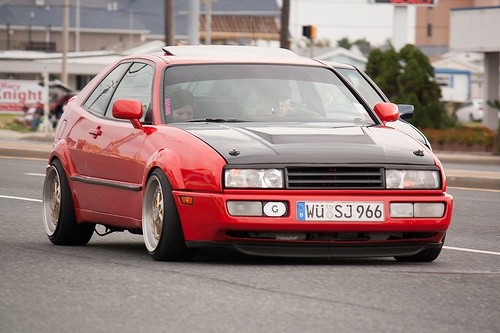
165,89 -> 194,123
255,78 -> 291,119
4,102 -> 43,130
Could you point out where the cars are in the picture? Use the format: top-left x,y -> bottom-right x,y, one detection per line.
452,100 -> 484,124
326,61 -> 432,152
41,45 -> 453,261
